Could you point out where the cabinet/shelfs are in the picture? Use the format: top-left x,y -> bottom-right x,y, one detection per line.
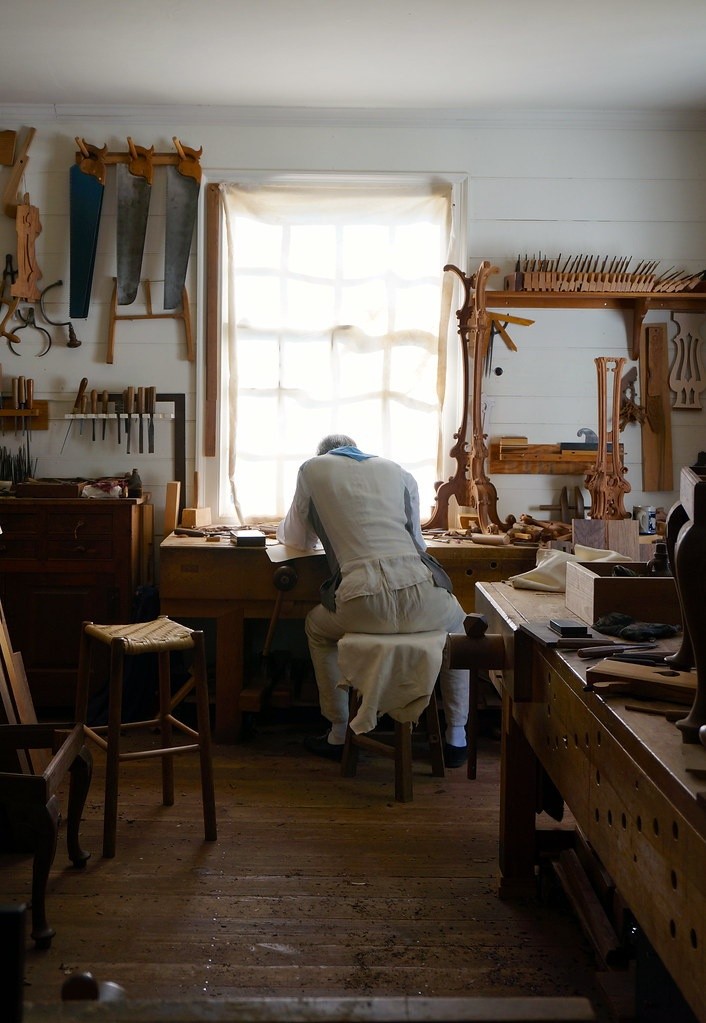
1,492 -> 151,706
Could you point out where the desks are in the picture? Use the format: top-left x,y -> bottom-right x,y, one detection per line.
160,525 -> 547,743
472,581 -> 706,1023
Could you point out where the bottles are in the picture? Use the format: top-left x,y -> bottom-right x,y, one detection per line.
128,469 -> 142,498
646,544 -> 673,576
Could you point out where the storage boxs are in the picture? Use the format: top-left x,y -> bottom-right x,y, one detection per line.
564,561 -> 681,628
230,530 -> 266,546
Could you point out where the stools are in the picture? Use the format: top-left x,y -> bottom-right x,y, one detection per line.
76,615 -> 217,858
338,630 -> 453,802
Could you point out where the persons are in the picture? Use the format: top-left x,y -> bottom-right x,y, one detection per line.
277,434 -> 477,767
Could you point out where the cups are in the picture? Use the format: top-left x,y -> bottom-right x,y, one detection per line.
634,505 -> 656,534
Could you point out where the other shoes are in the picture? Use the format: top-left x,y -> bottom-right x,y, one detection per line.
304,734 -> 351,761
443,741 -> 468,770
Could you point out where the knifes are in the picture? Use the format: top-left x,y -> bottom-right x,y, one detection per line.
578,645 -> 656,658
558,638 -> 662,646
